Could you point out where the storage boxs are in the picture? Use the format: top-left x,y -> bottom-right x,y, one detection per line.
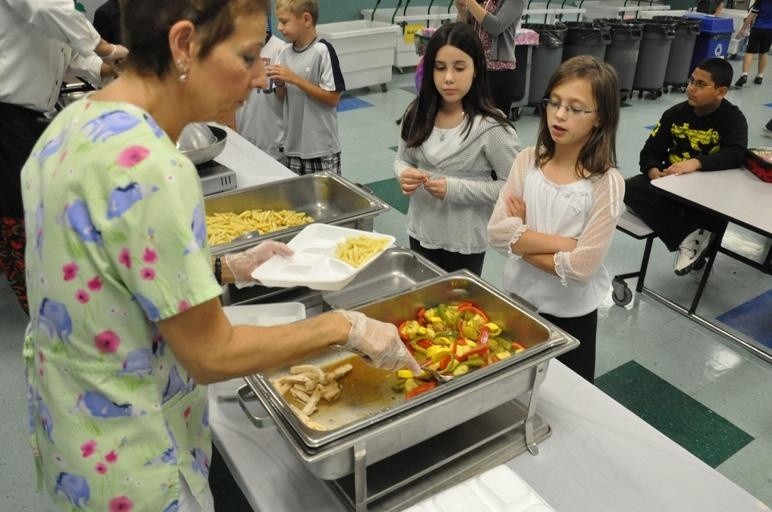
432,0 -> 459,28
719,9 -> 751,57
633,0 -> 671,20
550,0 -> 587,25
576,0 -> 642,23
312,0 -> 402,94
360,0 -> 439,74
520,0 -> 555,25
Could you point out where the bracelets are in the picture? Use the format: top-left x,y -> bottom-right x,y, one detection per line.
273,82 -> 285,88
215,256 -> 221,285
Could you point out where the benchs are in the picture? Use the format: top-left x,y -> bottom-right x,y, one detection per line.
612,206 -> 657,306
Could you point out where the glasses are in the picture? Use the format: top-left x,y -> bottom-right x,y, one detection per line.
685,78 -> 716,91
542,97 -> 596,119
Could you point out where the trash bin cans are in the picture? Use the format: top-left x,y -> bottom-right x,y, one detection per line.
509,14 -> 735,122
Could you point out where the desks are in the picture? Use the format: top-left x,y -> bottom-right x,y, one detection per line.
177,120 -> 771,512
643,146 -> 772,364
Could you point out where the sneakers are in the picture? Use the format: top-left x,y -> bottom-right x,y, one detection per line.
735,75 -> 749,88
754,75 -> 764,85
674,228 -> 717,277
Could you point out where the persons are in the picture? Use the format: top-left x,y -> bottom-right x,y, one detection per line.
735,0 -> 772,86
393,21 -> 519,278
453,0 -> 525,119
487,55 -> 626,383
696,0 -> 723,17
623,58 -> 747,277
19,0 -> 422,512
237,31 -> 286,160
264,0 -> 345,177
0,0 -> 130,318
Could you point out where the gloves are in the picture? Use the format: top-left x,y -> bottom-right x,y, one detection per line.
223,239 -> 296,289
332,307 -> 423,377
99,43 -> 130,75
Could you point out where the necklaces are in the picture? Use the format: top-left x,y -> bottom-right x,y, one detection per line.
437,111 -> 465,142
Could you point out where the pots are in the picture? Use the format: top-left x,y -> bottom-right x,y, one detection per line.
166,120 -> 231,168
